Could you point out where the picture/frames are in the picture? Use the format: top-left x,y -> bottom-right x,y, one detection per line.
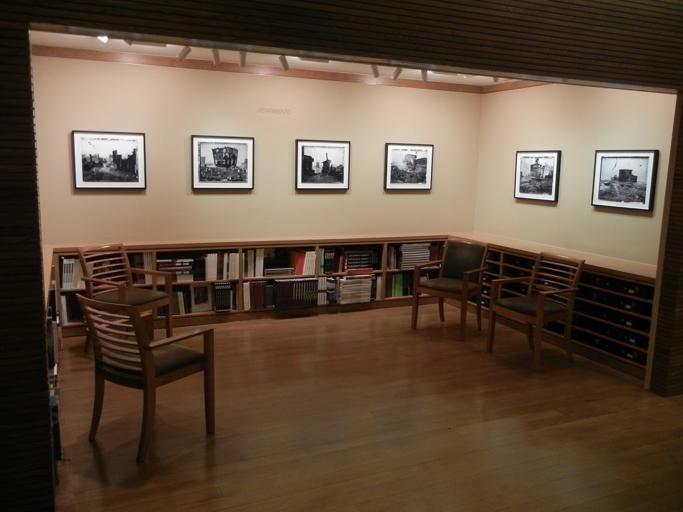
296,138 -> 350,190
384,142 -> 434,191
191,135 -> 255,190
71,130 -> 146,190
590,149 -> 660,212
513,150 -> 561,202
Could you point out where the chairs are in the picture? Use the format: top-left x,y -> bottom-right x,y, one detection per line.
75,294 -> 215,463
486,251 -> 586,370
410,236 -> 490,332
73,242 -> 177,354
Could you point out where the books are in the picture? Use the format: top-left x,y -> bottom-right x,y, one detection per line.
60,296 -> 67,326
229,253 -> 244,280
289,248 -> 316,276
319,248 -> 324,273
264,268 -> 294,275
338,256 -> 343,273
266,285 -> 274,308
223,252 -> 228,280
243,281 -> 265,311
287,280 -> 319,305
371,276 -> 381,300
214,282 -> 233,312
191,285 -> 212,312
171,291 -> 185,315
326,276 -> 340,304
190,253 -> 217,281
339,274 -> 372,304
247,250 -> 254,277
343,250 -> 373,273
324,250 -> 337,273
155,258 -> 194,275
255,249 -> 264,276
62,259 -> 93,289
387,243 -> 430,270
386,271 -> 410,297
317,277 -> 327,305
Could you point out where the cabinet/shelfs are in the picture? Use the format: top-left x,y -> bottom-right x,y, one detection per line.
447,233 -> 657,381
42,233 -> 447,486
